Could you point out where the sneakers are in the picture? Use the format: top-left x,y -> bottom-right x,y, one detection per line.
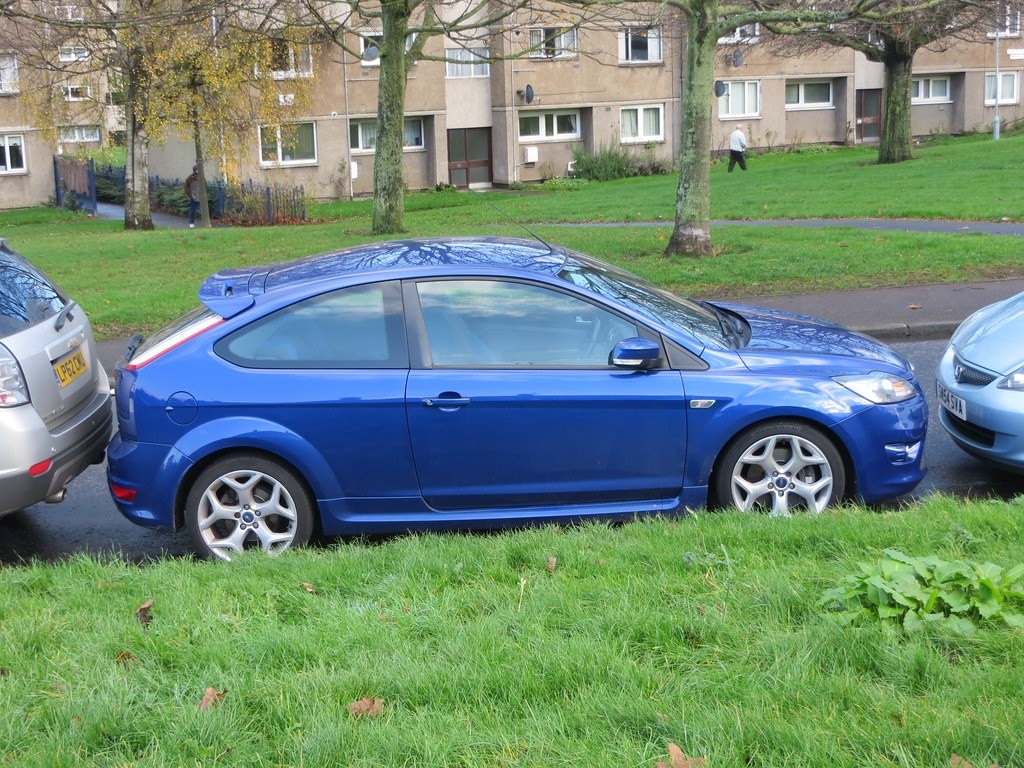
187,223 -> 194,227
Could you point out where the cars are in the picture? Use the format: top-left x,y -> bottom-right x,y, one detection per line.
102,237 -> 932,565
0,236 -> 113,520
933,291 -> 1024,477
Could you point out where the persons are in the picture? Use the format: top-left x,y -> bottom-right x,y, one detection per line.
728,124 -> 748,172
185,165 -> 213,229
